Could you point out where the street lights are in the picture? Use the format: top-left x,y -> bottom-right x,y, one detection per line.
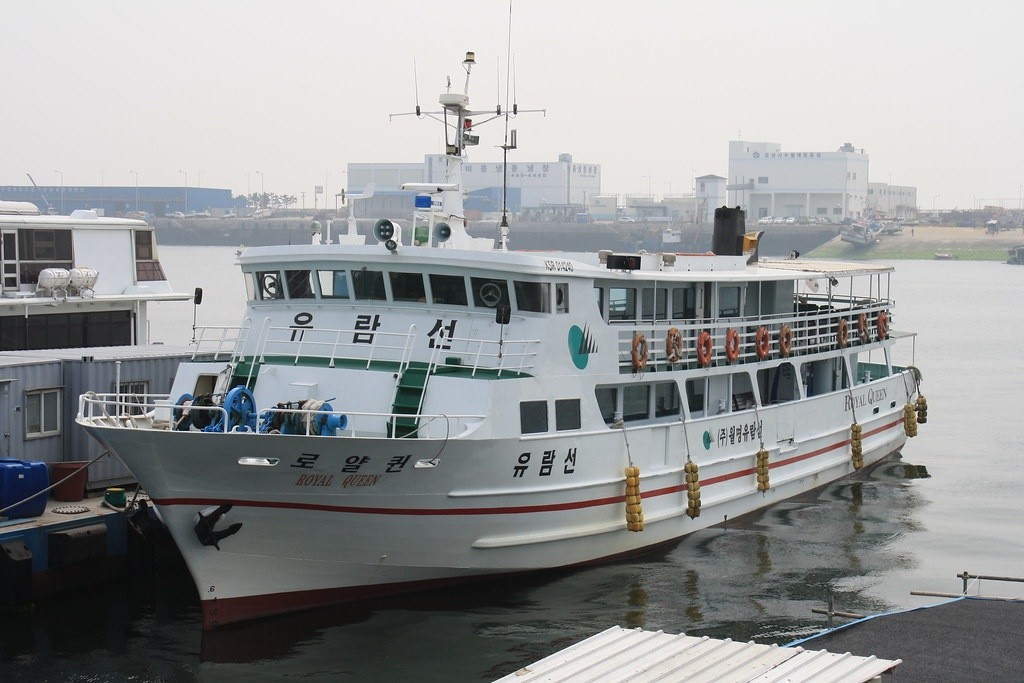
835,203 -> 842,221
256,170 -> 265,218
130,169 -> 139,212
179,170 -> 188,215
55,169 -> 64,214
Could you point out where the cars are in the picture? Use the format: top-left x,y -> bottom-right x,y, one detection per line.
618,217 -> 634,224
785,218 -> 796,224
758,216 -> 773,224
773,217 -> 785,224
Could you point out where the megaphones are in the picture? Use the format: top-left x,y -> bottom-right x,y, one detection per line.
434,222 -> 455,238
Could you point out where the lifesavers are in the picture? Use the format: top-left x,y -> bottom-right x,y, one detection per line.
859,312 -> 869,341
838,319 -> 848,346
756,325 -> 770,359
779,325 -> 793,354
665,326 -> 683,361
878,312 -> 889,338
634,334 -> 648,369
699,331 -> 712,363
726,329 -> 740,359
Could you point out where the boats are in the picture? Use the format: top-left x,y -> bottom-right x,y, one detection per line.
73,43 -> 928,632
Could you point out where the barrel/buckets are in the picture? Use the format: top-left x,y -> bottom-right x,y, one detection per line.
713,208 -> 745,256
0,457 -> 49,516
153,400 -> 172,422
50,464 -> 87,501
